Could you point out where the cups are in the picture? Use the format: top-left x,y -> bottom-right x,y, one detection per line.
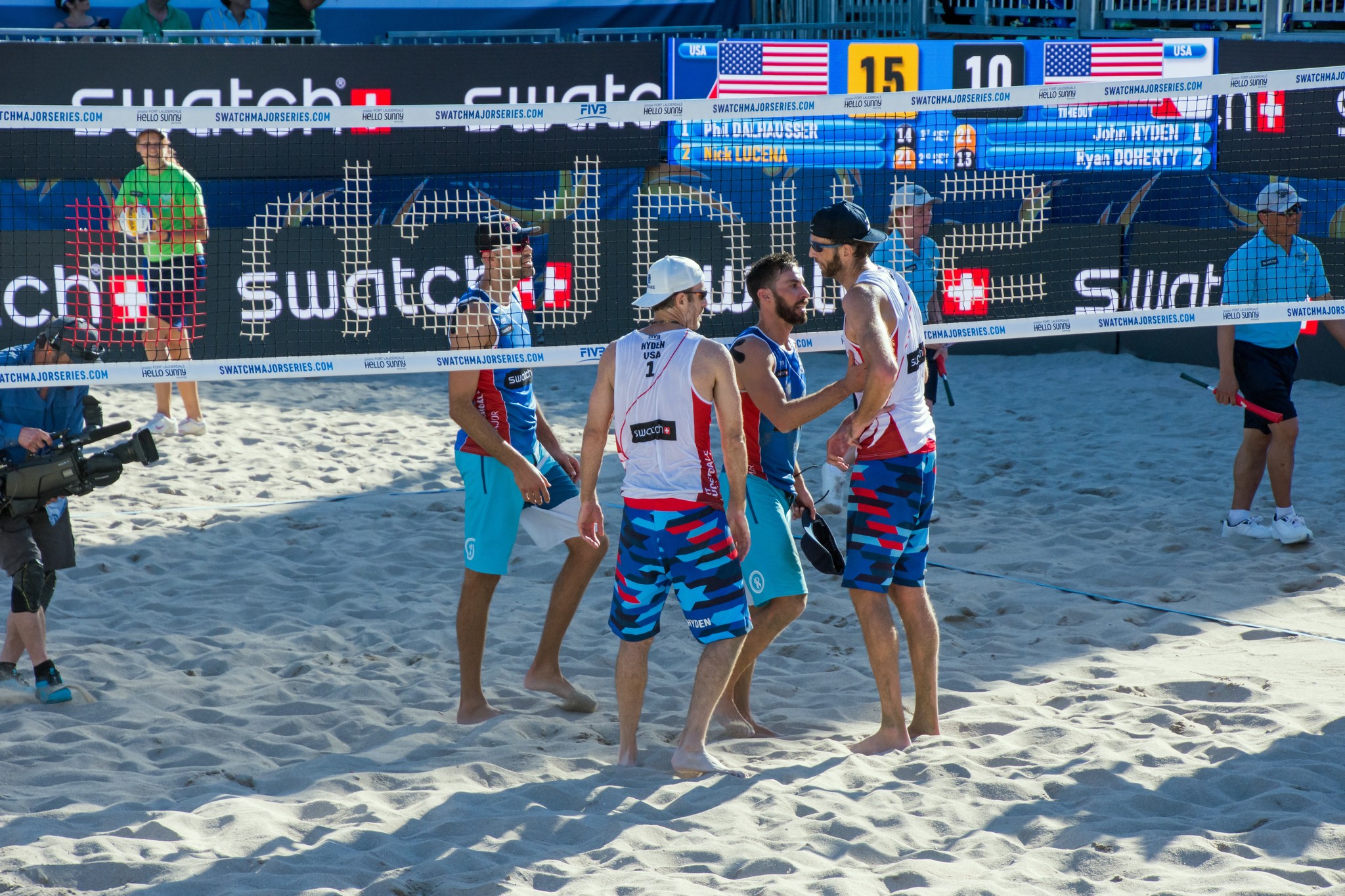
1213,20 -> 1228,31
1159,6 -> 1172,30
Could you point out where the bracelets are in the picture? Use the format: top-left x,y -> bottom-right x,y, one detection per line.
162,231 -> 172,243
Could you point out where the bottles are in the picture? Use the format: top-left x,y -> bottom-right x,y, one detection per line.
1113,22 -> 1138,30
1192,22 -> 1217,31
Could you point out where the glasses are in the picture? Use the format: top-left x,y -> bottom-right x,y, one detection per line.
480,234 -> 529,254
793,465 -> 830,509
1261,203 -> 1302,216
59,337 -> 104,360
137,142 -> 164,151
669,288 -> 707,300
809,236 -> 856,252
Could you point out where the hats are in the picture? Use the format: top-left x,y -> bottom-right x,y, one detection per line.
1256,182 -> 1307,212
475,214 -> 542,250
45,316 -> 100,364
890,184 -> 943,212
631,255 -> 705,308
810,200 -> 888,243
800,508 -> 845,575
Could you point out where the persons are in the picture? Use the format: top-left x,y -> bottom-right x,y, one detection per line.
263,0 -> 326,44
715,250 -> 871,736
870,185 -> 948,521
107,128 -> 210,436
120,0 -> 197,44
1215,184 -> 1345,545
808,200 -> 940,757
578,254 -> 755,777
200,0 -> 267,44
50,0 -> 116,43
446,213 -> 611,725
0,315 -> 106,704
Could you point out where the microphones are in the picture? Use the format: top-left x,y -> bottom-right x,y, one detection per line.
52,421 -> 132,450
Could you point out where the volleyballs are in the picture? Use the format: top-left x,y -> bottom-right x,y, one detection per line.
119,202 -> 157,240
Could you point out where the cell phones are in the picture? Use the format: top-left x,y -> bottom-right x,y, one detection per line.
97,19 -> 109,27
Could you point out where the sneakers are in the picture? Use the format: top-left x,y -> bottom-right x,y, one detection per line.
34,668 -> 73,705
929,511 -> 941,522
0,665 -> 30,686
821,462 -> 847,515
137,412 -> 178,435
1220,515 -> 1273,539
179,417 -> 206,435
1271,512 -> 1313,545
